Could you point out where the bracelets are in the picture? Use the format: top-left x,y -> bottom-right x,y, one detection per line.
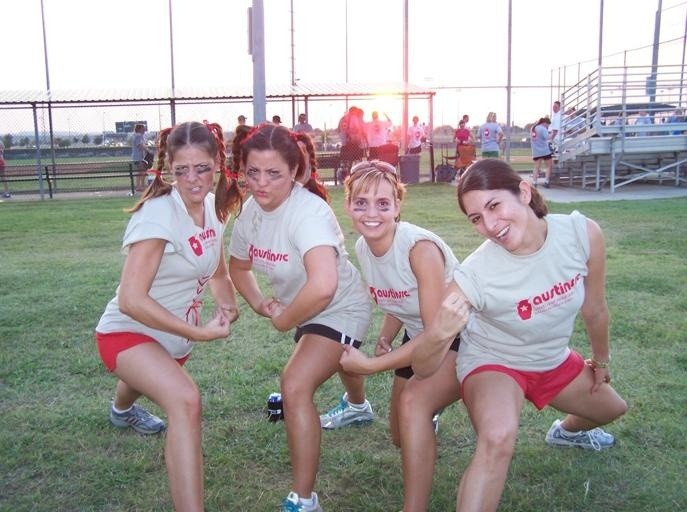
590,355 -> 610,369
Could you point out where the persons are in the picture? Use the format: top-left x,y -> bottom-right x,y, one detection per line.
530,118 -> 554,188
407,156 -> 632,511
407,116 -> 425,153
453,115 -> 474,155
477,112 -> 504,158
614,110 -> 629,137
92,122 -> 240,511
131,123 -> 151,192
550,101 -> 585,143
340,159 -> 466,512
272,116 -> 282,124
0,141 -> 11,199
634,106 -> 652,137
238,115 -> 247,126
665,106 -> 687,135
337,106 -> 393,183
228,124 -> 377,511
293,114 -> 316,138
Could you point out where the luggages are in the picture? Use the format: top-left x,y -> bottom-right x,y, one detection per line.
436,143 -> 453,182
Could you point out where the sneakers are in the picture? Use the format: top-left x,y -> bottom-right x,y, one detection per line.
544,418 -> 614,451
281,491 -> 322,512
319,392 -> 373,428
110,401 -> 166,435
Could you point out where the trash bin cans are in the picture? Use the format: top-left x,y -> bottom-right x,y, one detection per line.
398,154 -> 421,185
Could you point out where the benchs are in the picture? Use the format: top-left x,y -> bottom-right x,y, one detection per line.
537,121 -> 687,195
0,160 -> 147,201
310,152 -> 346,186
